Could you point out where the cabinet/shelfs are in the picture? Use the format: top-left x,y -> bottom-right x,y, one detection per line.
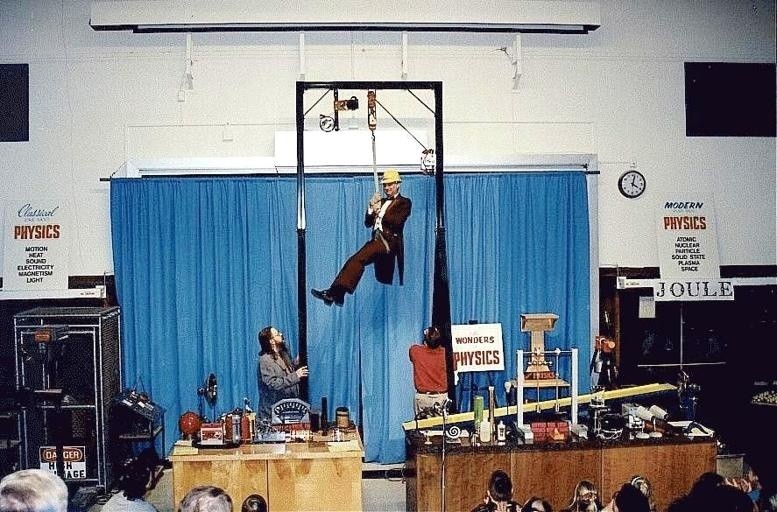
614,280 -> 734,449
405,417 -> 720,512
10,301 -> 124,496
162,431 -> 369,511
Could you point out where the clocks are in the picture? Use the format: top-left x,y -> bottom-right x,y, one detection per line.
618,170 -> 647,199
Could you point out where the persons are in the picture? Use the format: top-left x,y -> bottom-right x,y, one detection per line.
409,327 -> 457,419
0,469 -> 69,512
311,170 -> 413,307
178,485 -> 233,512
99,457 -> 160,512
241,494 -> 267,512
255,326 -> 310,423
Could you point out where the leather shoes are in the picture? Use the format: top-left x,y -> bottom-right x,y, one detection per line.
311,286 -> 344,306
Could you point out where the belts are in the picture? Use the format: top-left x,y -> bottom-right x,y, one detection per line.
417,390 -> 448,394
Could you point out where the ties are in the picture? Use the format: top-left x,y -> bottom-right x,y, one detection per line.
278,350 -> 293,374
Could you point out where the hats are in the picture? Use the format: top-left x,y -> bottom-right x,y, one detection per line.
380,170 -> 402,183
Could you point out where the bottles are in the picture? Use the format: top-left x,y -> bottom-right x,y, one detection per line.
240,414 -> 249,441
225,414 -> 233,439
497,420 -> 506,441
481,417 -> 492,443
336,407 -> 350,429
474,395 -> 485,431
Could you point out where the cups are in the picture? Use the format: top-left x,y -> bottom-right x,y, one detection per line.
311,414 -> 319,432
589,385 -> 606,405
